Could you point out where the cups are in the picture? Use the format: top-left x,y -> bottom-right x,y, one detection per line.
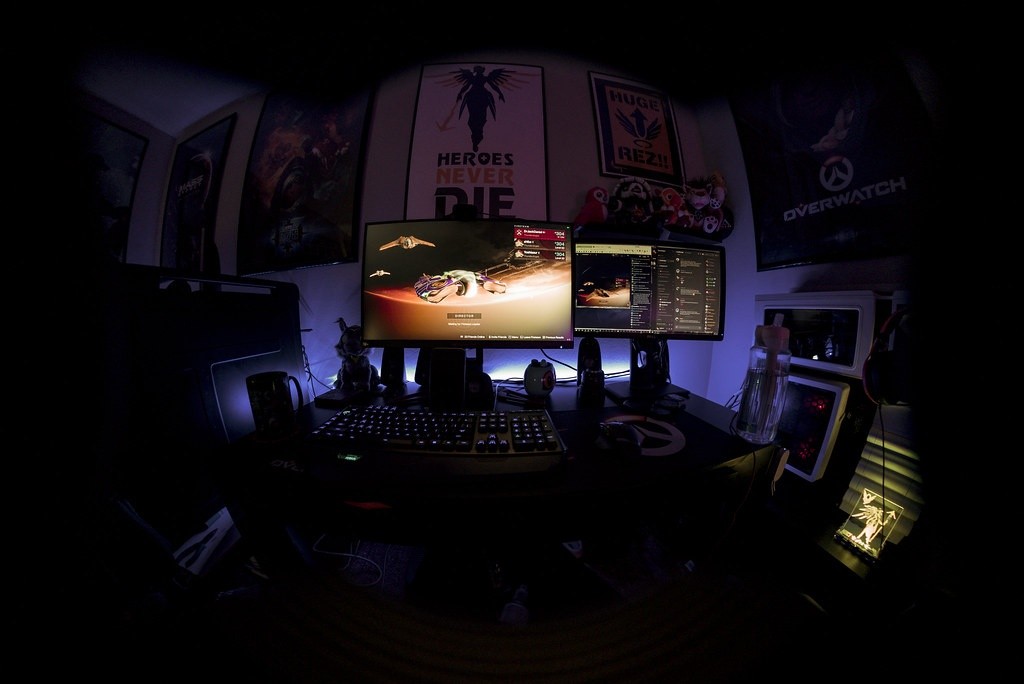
246,371 -> 304,433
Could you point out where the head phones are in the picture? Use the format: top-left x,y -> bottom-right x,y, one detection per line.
862,309 -> 913,406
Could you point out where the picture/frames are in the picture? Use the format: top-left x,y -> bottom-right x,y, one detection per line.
84,113 -> 150,263
588,70 -> 687,188
236,62 -> 379,277
403,61 -> 549,222
767,372 -> 851,483
159,113 -> 238,271
752,288 -> 878,380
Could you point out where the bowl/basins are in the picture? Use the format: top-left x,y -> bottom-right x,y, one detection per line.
524,362 -> 556,396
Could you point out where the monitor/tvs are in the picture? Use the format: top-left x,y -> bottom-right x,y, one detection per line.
360,218 -> 575,411
573,237 -> 726,402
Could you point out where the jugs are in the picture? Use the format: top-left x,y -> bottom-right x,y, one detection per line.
736,313 -> 792,444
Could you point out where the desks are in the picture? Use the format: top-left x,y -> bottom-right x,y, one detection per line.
107,260 -> 926,616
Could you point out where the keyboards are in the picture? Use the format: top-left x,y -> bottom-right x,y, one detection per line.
304,405 -> 567,474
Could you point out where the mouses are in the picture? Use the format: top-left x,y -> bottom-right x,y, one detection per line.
600,417 -> 642,458
